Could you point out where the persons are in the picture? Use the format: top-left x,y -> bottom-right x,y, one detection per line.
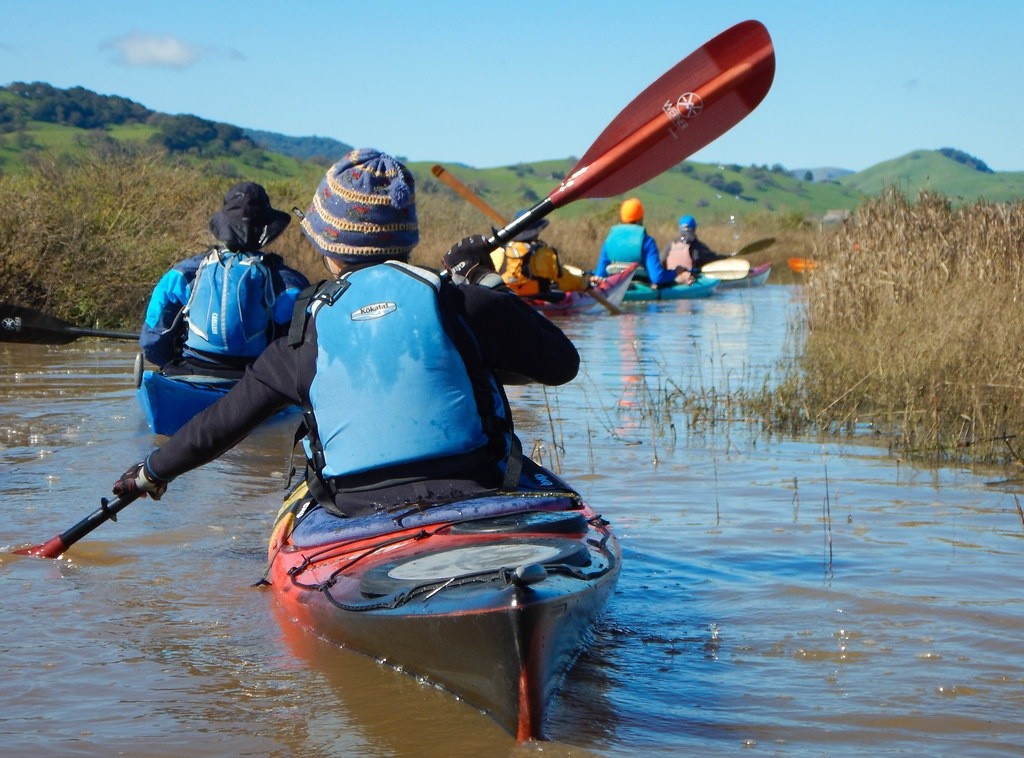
140,179 -> 308,382
485,198 -> 732,293
112,148 -> 579,518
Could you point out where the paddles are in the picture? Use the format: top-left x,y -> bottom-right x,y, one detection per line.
6,18 -> 779,562
562,236 -> 821,281
0,301 -> 142,347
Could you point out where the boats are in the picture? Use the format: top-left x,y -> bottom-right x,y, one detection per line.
132,351 -> 303,446
521,261 -> 641,317
786,256 -> 822,275
622,265 -> 724,302
268,455 -> 625,744
719,262 -> 775,289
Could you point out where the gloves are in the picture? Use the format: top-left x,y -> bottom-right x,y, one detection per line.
112,463 -> 167,501
440,234 -> 500,285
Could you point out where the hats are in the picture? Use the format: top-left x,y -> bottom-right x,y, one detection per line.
678,215 -> 696,228
621,198 -> 643,222
209,181 -> 291,252
299,147 -> 419,262
510,209 -> 549,242
